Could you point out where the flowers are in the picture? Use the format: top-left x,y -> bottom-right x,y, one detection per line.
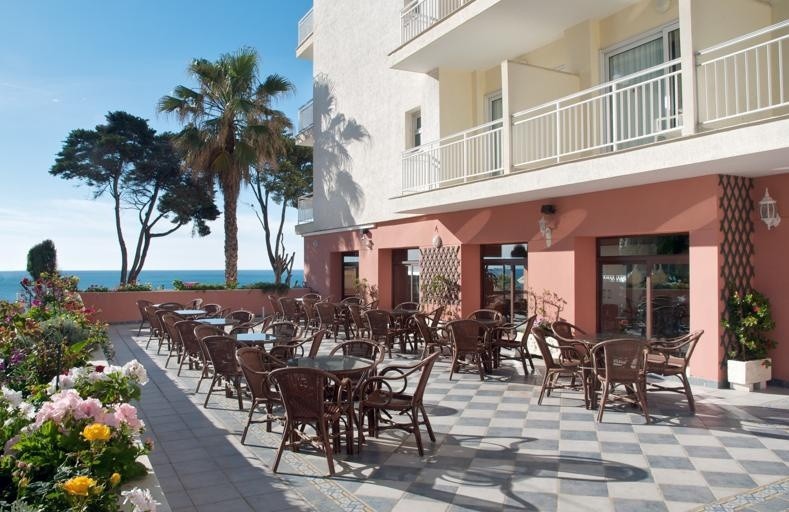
723,288 -> 777,369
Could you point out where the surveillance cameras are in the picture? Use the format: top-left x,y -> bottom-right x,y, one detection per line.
358,228 -> 369,235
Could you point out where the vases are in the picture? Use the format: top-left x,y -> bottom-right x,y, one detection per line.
728,360 -> 771,391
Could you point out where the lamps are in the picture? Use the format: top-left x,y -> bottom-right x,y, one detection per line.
360,229 -> 372,248
540,205 -> 555,247
759,187 -> 781,230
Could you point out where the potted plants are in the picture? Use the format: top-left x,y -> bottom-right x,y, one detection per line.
517,291 -> 566,358
420,275 -> 460,335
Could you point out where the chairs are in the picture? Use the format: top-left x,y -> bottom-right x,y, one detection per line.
616,297 -> 688,336
655,114 -> 682,142
444,310 -> 704,423
